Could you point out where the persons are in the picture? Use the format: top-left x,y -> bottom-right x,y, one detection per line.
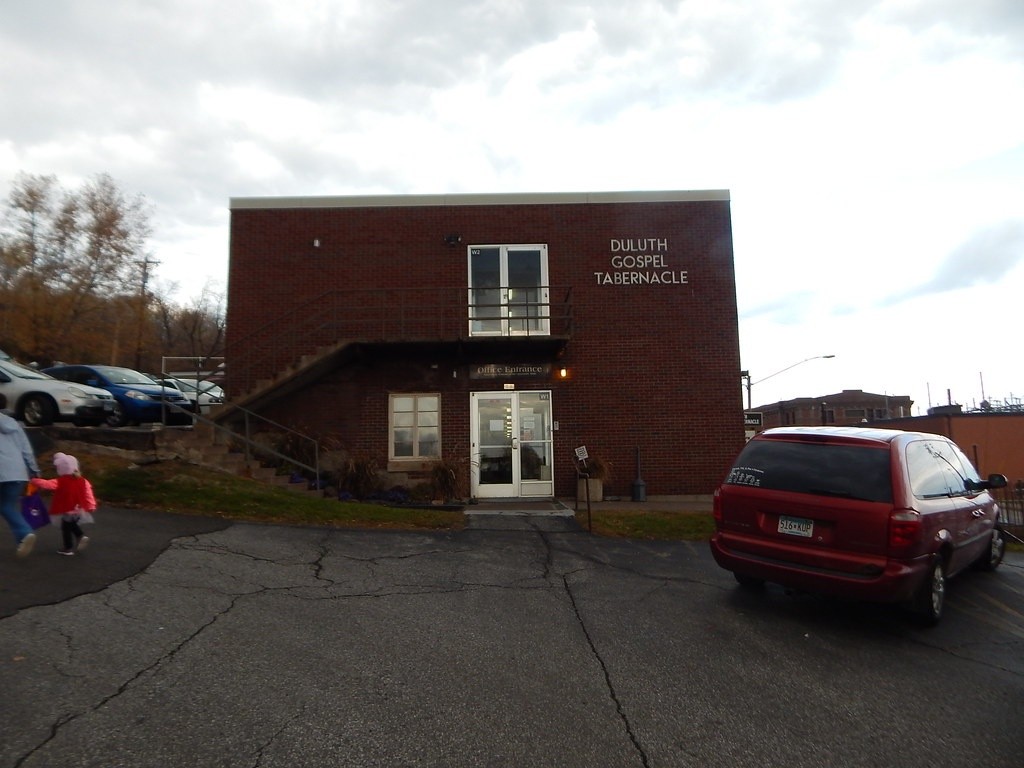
0,393 -> 96,557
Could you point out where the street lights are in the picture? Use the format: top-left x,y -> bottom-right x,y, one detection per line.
747,355 -> 836,410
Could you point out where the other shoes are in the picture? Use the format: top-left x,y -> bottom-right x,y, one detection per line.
17,533 -> 36,558
76,535 -> 89,550
56,548 -> 74,555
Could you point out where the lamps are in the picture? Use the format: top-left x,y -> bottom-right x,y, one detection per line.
441,230 -> 465,247
311,235 -> 325,248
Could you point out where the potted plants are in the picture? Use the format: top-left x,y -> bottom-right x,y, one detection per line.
572,446 -> 613,501
425,458 -> 480,504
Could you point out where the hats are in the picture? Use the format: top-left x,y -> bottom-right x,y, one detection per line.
30,362 -> 39,366
53,452 -> 80,475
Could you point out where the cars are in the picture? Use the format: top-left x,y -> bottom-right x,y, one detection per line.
710,424 -> 1008,627
0,357 -> 118,427
155,379 -> 225,415
41,365 -> 193,426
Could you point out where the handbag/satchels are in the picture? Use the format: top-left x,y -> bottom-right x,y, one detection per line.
21,493 -> 51,529
